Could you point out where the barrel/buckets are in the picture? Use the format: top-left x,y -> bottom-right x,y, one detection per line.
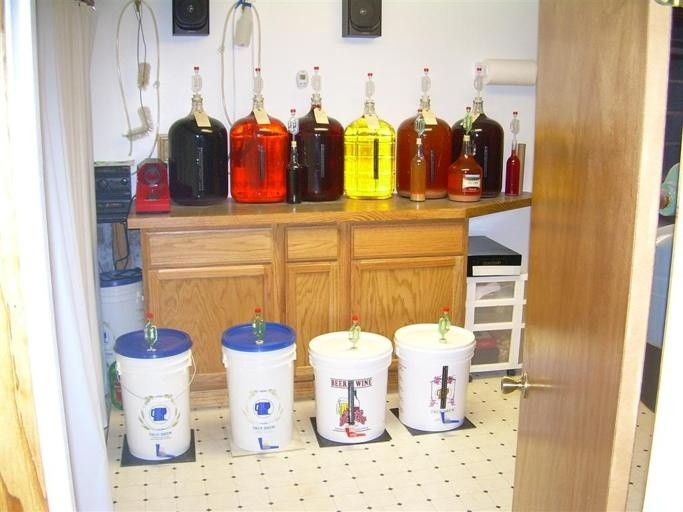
220,322 -> 297,452
394,324 -> 477,432
100,268 -> 144,382
112,329 -> 197,461
307,330 -> 393,444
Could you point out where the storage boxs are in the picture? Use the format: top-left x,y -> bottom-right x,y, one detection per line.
469,235 -> 523,274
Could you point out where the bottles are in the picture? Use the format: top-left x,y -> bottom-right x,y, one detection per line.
448,134 -> 483,201
286,141 -> 302,203
143,311 -> 157,352
348,315 -> 361,350
411,137 -> 427,204
251,307 -> 264,344
437,306 -> 451,342
504,134 -> 520,197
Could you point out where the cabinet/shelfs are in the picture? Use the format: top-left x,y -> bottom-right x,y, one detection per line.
464,276 -> 530,374
347,219 -> 465,374
145,229 -> 277,392
285,225 -> 344,379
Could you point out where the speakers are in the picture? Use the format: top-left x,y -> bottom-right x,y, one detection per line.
342,0 -> 381,38
172,0 -> 209,36
